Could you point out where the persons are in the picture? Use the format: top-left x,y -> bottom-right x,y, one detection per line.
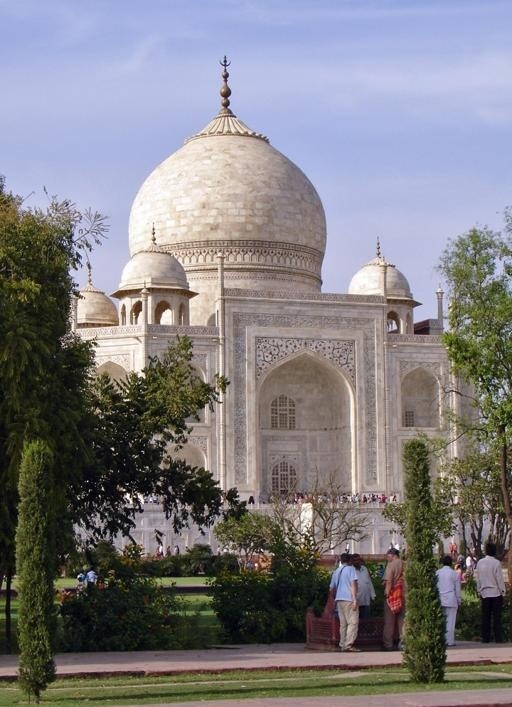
153,541 -> 237,560
96,575 -> 106,589
86,568 -> 99,587
279,491 -> 398,508
449,541 -> 486,586
435,554 -> 463,647
77,572 -> 86,588
108,573 -> 115,584
350,554 -> 377,646
329,553 -> 362,652
382,548 -> 406,651
475,543 -> 509,644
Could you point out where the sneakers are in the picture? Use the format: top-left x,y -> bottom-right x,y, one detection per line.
343,646 -> 361,652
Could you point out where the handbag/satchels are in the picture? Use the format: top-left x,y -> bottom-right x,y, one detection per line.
386,581 -> 404,614
